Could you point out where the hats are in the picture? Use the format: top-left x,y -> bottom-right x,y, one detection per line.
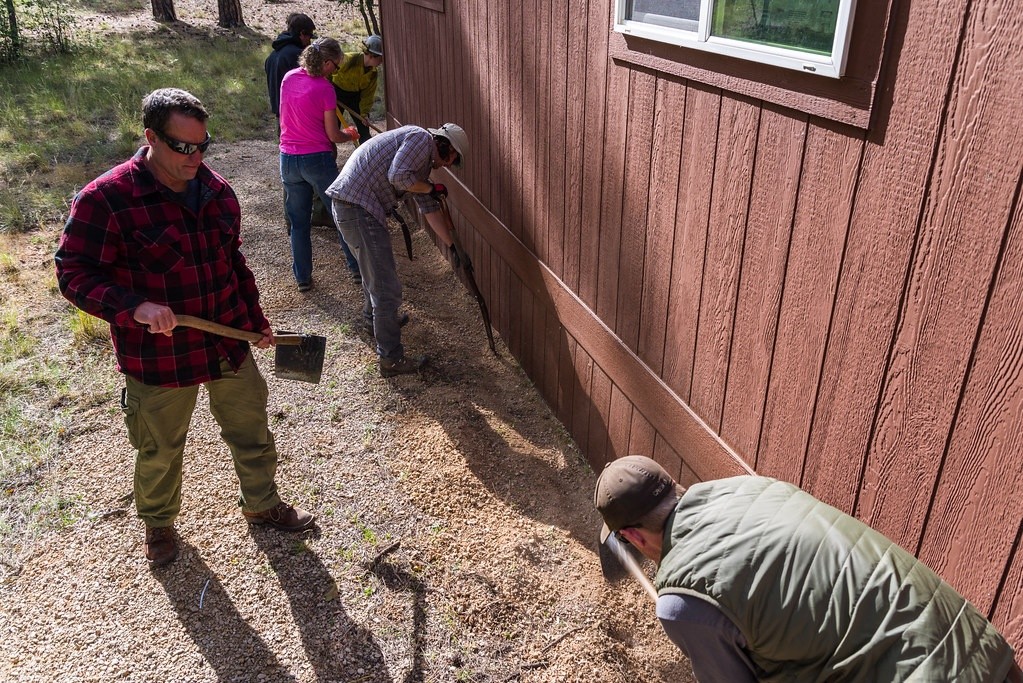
593,455 -> 676,544
427,122 -> 469,170
362,35 -> 382,55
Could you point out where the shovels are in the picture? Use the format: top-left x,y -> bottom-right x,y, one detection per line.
436,192 -> 495,353
336,106 -> 413,262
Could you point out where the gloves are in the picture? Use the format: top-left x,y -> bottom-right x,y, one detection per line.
429,184 -> 449,201
343,125 -> 360,139
450,243 -> 473,271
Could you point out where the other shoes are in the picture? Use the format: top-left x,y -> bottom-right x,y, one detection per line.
352,273 -> 362,284
297,279 -> 314,291
311,210 -> 335,228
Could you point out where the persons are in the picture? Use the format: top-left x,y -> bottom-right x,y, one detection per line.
330,36 -> 384,178
326,122 -> 471,379
592,455 -> 1023,683
265,12 -> 319,145
56,88 -> 317,570
278,35 -> 362,292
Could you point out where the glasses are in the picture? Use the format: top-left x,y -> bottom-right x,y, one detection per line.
152,126 -> 212,155
324,58 -> 340,72
307,31 -> 318,39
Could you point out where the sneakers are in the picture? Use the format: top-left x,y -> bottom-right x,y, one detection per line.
379,356 -> 418,378
142,522 -> 177,564
243,500 -> 314,531
363,315 -> 409,337
